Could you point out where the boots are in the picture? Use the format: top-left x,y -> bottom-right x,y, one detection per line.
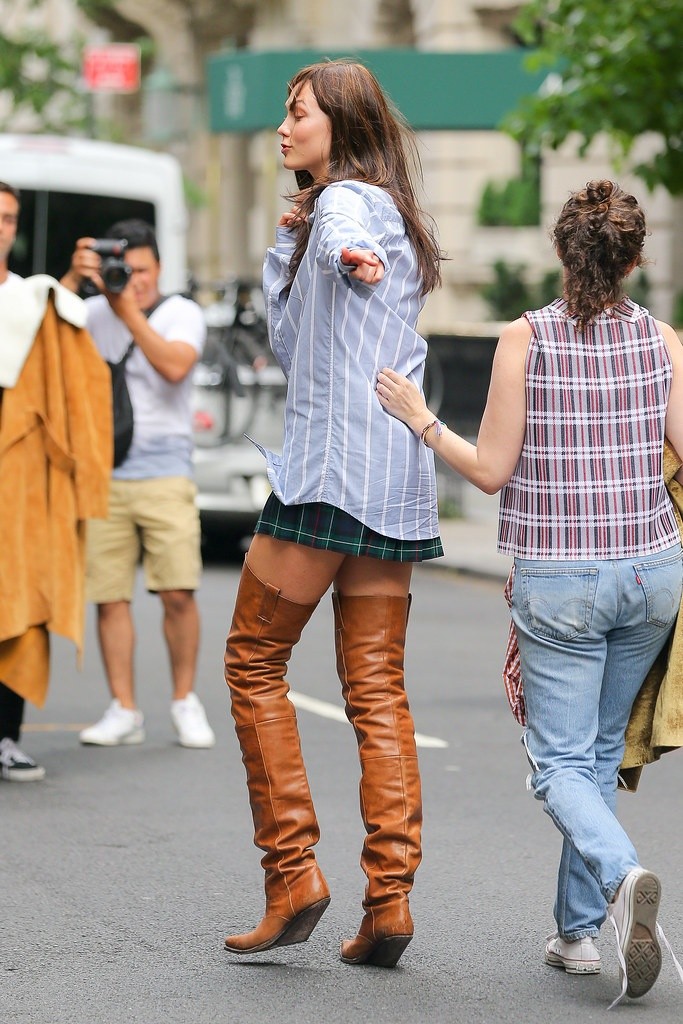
221,556 -> 330,954
333,591 -> 422,971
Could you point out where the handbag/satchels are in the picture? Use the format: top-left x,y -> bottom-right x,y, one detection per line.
106,296 -> 171,468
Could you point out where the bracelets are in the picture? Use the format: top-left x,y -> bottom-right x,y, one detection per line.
421,422 -> 445,447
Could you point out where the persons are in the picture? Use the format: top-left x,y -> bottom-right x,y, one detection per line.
0,182 -> 114,782
225,62 -> 442,966
59,219 -> 214,746
375,182 -> 682,1009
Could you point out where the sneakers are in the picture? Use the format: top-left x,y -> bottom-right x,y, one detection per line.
171,695 -> 218,749
79,698 -> 147,749
0,738 -> 47,782
545,939 -> 602,978
616,870 -> 664,1001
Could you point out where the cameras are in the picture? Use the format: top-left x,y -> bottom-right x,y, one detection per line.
90,239 -> 129,293
